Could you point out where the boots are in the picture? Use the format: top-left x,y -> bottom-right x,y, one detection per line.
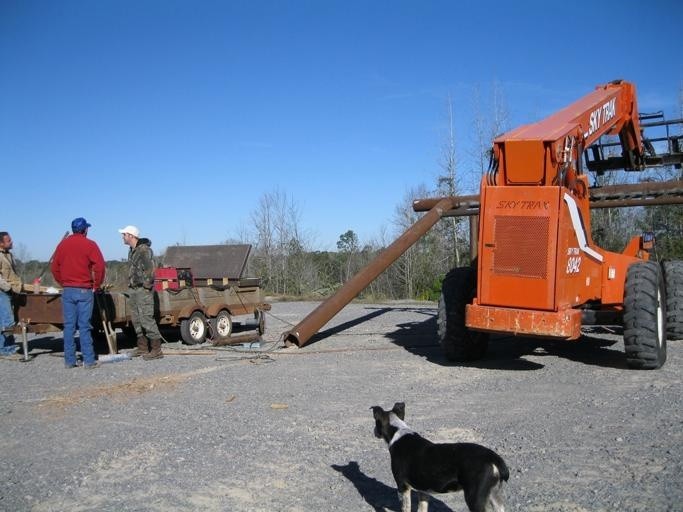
144,340 -> 162,359
126,337 -> 149,356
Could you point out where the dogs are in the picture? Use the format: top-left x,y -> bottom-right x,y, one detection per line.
369,402 -> 510,512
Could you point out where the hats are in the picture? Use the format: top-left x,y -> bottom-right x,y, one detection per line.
118,226 -> 139,238
71,217 -> 91,228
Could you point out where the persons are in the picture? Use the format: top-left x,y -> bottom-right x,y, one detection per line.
51,218 -> 107,369
0,232 -> 23,360
119,226 -> 164,360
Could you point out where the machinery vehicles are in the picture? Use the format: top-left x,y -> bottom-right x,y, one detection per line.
433,77 -> 682,372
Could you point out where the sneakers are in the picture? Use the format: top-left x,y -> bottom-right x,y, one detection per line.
85,363 -> 100,368
0,352 -> 23,360
